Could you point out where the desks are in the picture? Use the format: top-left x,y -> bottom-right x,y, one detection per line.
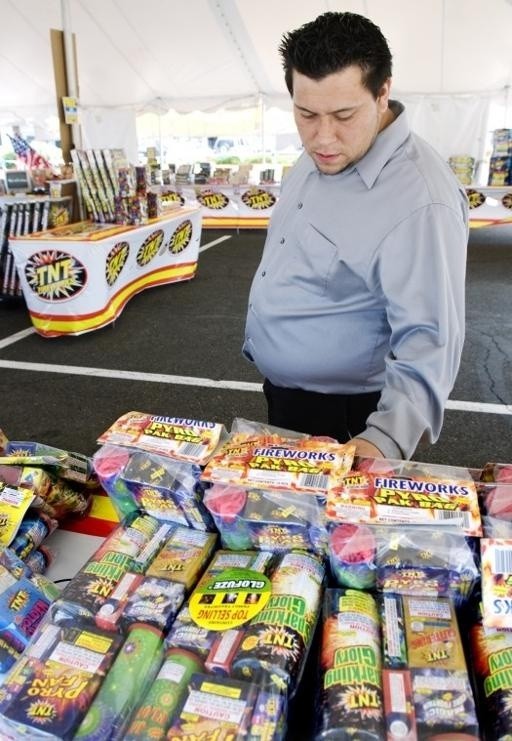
147,183 -> 280,236
463,186 -> 512,229
11,204 -> 204,339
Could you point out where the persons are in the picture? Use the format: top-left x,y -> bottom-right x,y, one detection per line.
242,10 -> 468,460
